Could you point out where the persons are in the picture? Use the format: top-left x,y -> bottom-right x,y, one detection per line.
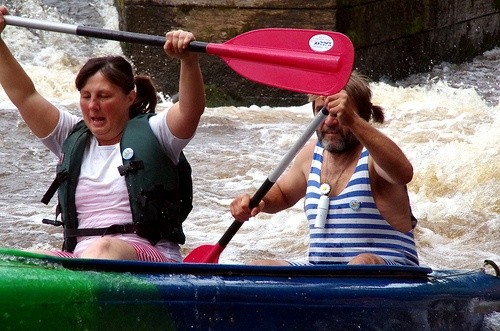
229,71 -> 418,267
0,5 -> 205,262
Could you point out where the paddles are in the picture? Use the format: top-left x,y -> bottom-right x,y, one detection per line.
183,108 -> 329,263
3,14 -> 354,96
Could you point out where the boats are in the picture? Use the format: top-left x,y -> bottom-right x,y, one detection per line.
0,250 -> 500,331
113,1 -> 499,105
